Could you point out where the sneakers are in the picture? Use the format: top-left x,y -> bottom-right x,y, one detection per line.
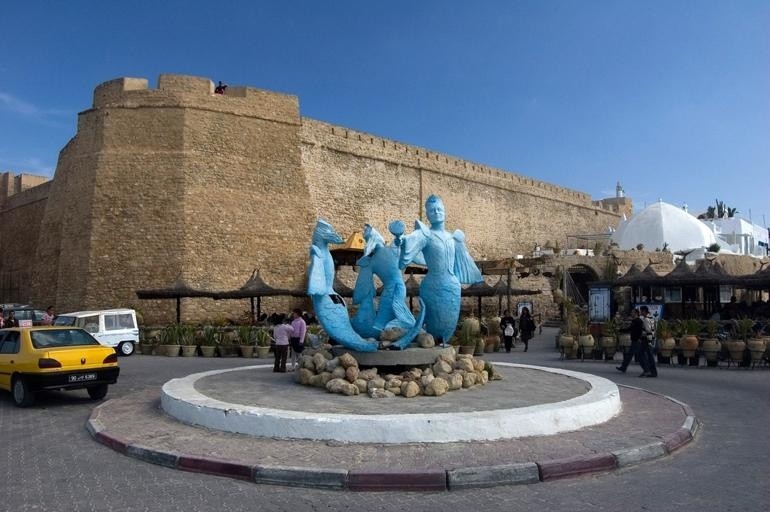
288,367 -> 295,372
616,366 -> 657,377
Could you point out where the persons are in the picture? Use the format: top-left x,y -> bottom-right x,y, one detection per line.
518,306 -> 535,352
394,194 -> 482,346
40,306 -> 55,326
215,81 -> 227,94
685,294 -> 770,319
615,308 -> 652,377
636,305 -> 658,378
289,308 -> 307,372
5,310 -> 19,326
259,311 -> 317,325
500,310 -> 516,353
272,314 -> 295,373
0,308 -> 5,329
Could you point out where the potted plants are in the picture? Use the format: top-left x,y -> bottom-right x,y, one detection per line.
560,313 -> 770,361
138,325 -> 271,359
450,329 -> 499,355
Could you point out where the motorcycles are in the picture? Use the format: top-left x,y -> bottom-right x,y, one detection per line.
50,309 -> 142,357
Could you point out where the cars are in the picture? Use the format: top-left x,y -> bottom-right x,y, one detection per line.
0,326 -> 120,408
0,301 -> 72,326
